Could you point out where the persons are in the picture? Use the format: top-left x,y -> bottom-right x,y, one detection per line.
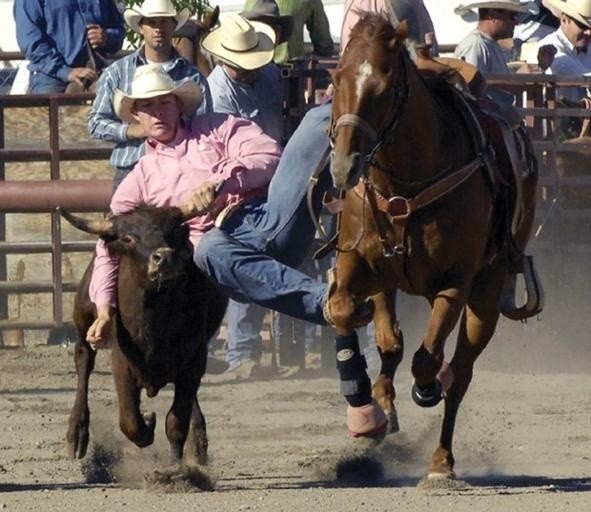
201,11 -> 308,380
340,1 -> 485,101
13,0 -> 126,95
452,0 -> 537,109
84,64 -> 375,351
86,0 -> 214,216
530,1 -> 591,142
245,4 -> 297,64
239,1 -> 339,65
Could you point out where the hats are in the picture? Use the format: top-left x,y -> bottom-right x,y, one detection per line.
454,0 -> 541,23
123,0 -> 191,35
113,63 -> 205,125
198,11 -> 277,71
541,1 -> 590,29
239,0 -> 295,46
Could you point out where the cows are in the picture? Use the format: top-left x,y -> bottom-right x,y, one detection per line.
56,179 -> 229,469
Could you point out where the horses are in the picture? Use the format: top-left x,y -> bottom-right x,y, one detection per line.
64,5 -> 220,95
329,5 -> 538,479
